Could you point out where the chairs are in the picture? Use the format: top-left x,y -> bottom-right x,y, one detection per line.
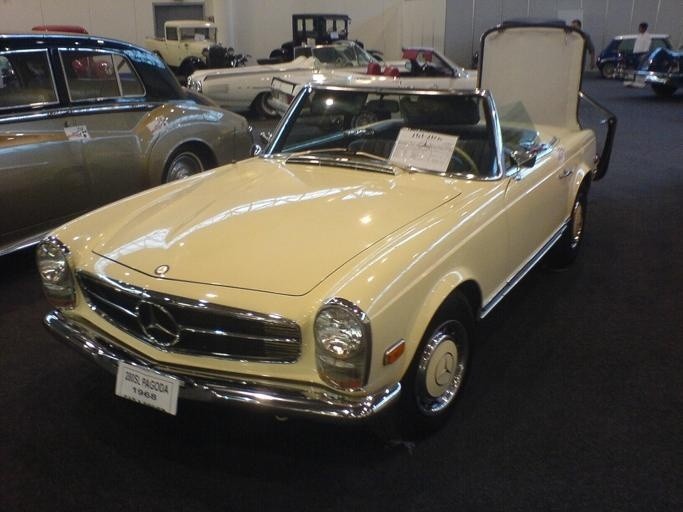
347,88 -> 495,175
366,60 -> 403,77
0,73 -> 145,106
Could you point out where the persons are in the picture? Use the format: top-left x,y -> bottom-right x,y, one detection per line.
570,19 -> 596,71
632,22 -> 652,73
